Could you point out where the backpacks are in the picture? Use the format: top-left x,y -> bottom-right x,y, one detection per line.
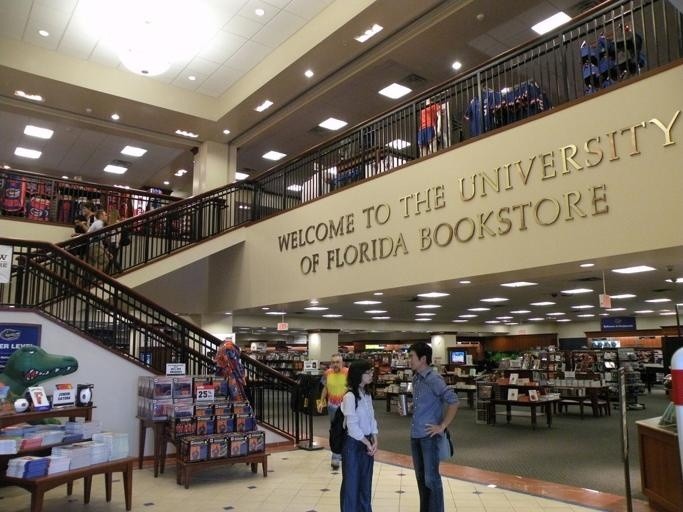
329,390 -> 359,454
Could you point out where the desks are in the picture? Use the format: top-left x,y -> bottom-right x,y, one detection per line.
0,404 -> 272,512
384,364 -> 611,429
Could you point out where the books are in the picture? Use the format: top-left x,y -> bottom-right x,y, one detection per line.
0,420 -> 129,479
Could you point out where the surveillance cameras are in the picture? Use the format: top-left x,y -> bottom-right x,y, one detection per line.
666,267 -> 673,272
552,293 -> 557,298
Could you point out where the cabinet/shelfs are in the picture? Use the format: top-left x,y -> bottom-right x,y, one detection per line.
256,359 -> 305,389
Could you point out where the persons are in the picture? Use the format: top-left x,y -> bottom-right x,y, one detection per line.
70,201 -> 123,288
407,342 -> 460,512
339,359 -> 379,512
417,98 -> 441,157
317,352 -> 349,471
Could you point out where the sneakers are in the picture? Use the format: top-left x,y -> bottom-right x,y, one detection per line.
331,458 -> 341,474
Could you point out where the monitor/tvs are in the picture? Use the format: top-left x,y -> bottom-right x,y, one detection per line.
450,350 -> 466,364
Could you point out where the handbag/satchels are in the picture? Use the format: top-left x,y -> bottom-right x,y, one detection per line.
436,428 -> 453,461
